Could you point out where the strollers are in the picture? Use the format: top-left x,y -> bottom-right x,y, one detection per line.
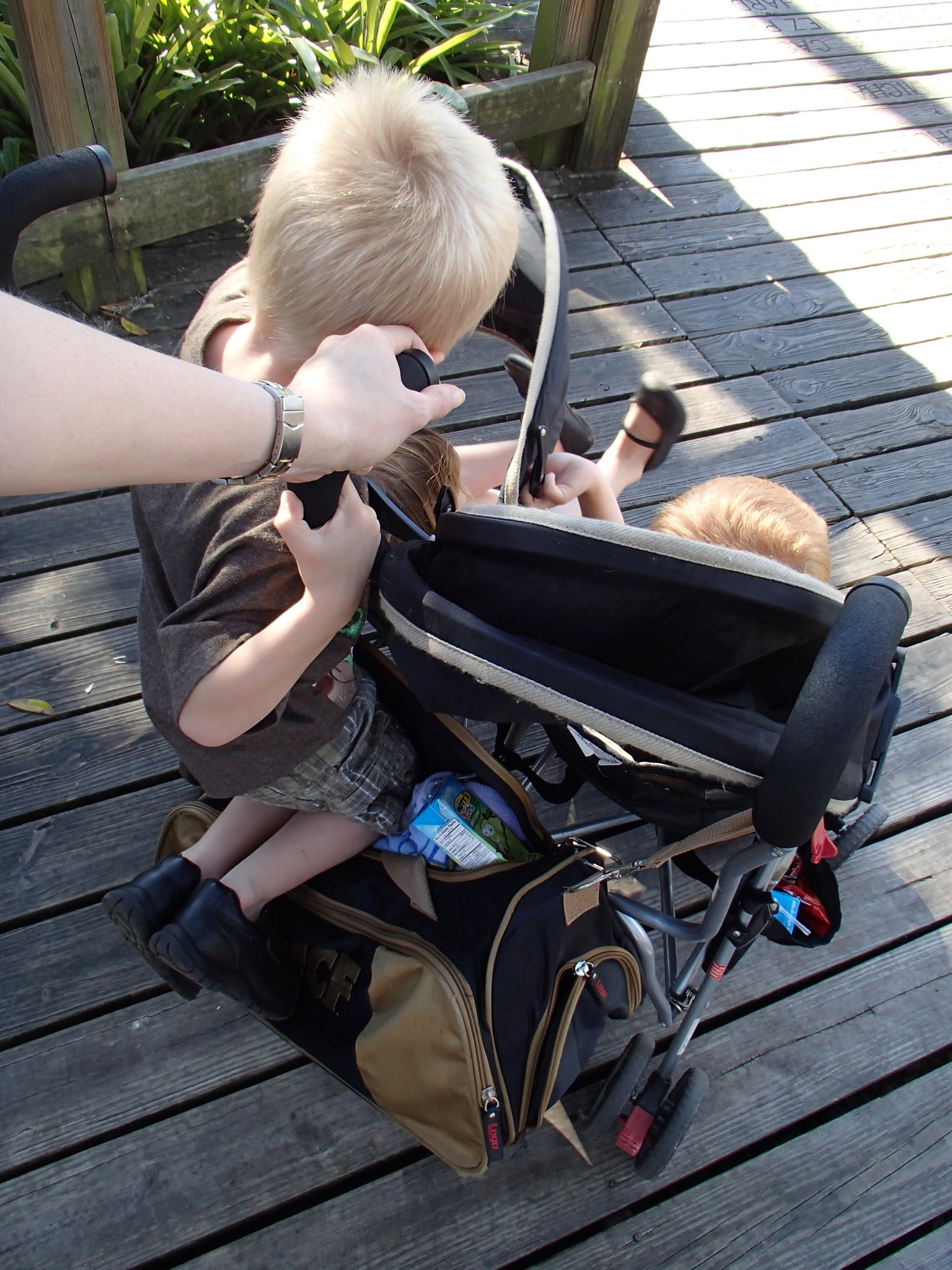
148,350 -> 921,1180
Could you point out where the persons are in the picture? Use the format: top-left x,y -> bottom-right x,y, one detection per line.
0,287 -> 467,487
104,67 -> 832,1003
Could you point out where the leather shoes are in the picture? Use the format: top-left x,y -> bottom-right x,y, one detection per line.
505,354 -> 594,457
624,371 -> 686,473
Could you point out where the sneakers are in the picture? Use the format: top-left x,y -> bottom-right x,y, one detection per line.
101,852 -> 202,1001
148,878 -> 293,1022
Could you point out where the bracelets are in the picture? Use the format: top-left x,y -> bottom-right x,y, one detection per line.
208,381 -> 304,486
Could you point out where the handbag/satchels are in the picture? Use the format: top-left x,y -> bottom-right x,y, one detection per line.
154,637 -> 647,1179
